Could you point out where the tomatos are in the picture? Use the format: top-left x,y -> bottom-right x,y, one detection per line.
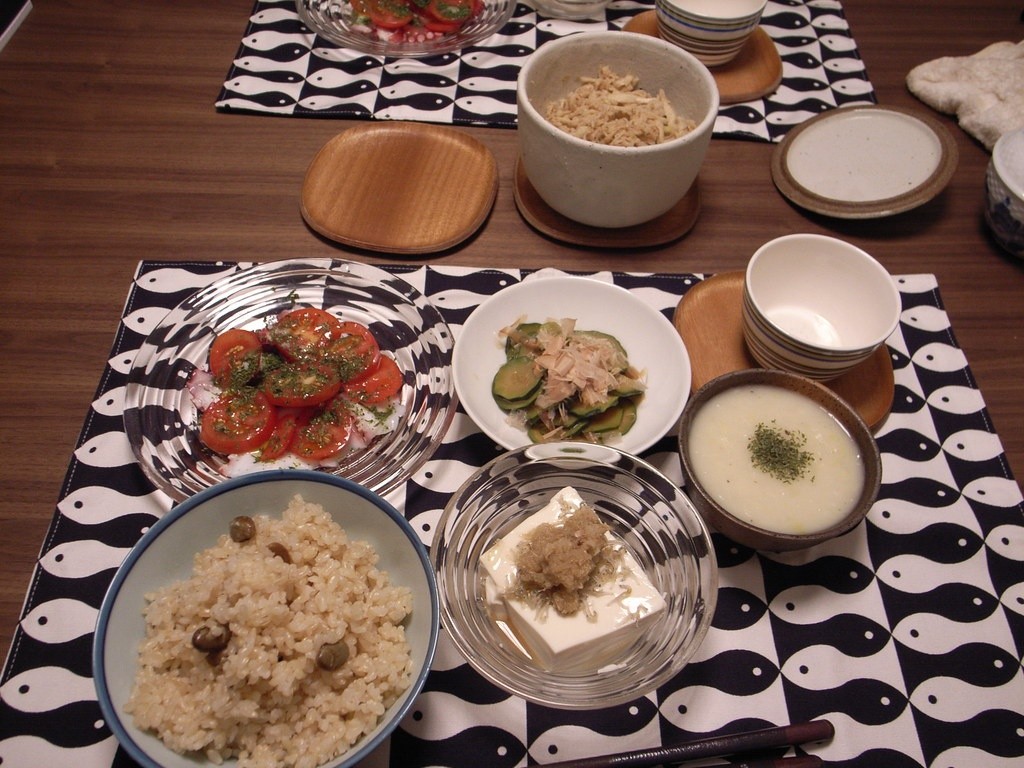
200,306 -> 403,463
351,0 -> 486,34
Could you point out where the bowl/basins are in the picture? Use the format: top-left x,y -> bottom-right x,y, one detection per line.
427,442 -> 721,710
516,30 -> 721,228
449,277 -> 691,470
676,367 -> 884,553
90,467 -> 440,768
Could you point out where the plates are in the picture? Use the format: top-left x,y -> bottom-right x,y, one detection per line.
120,256 -> 458,509
294,0 -> 518,58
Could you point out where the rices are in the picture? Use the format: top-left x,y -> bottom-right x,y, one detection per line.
121,493 -> 414,768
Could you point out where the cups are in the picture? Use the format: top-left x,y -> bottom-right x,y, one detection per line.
974,122 -> 1024,258
532,0 -> 611,18
653,0 -> 767,64
739,234 -> 904,381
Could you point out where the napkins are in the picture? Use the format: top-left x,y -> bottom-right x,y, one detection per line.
907,36 -> 1024,151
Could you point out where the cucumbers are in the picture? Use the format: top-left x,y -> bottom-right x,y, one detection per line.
492,322 -> 644,444
